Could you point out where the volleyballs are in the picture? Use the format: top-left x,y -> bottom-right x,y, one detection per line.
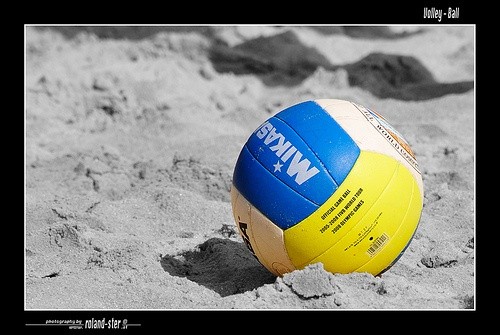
230,99 -> 423,278
